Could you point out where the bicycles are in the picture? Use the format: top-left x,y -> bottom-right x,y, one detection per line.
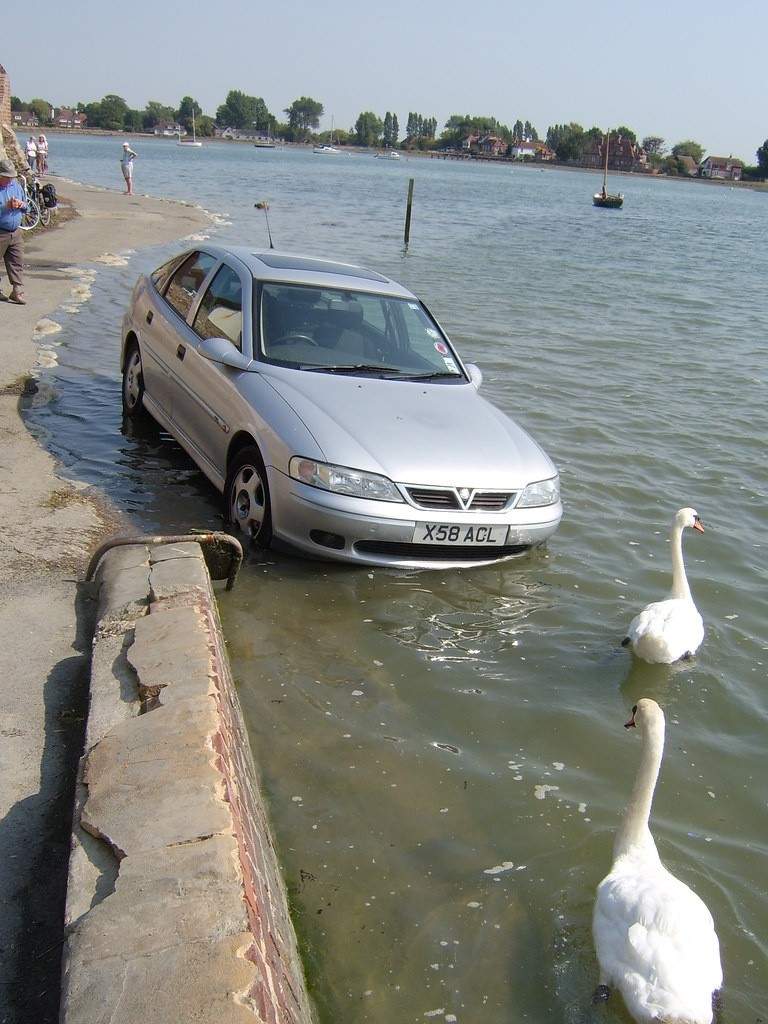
15,168 -> 52,231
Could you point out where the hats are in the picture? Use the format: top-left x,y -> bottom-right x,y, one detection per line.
0,160 -> 16,177
123,143 -> 129,145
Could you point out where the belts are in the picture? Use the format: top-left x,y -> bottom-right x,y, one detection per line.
1,227 -> 17,233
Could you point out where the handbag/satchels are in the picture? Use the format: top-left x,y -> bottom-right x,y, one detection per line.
43,184 -> 57,207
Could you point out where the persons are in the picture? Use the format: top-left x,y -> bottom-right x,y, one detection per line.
0,159 -> 30,304
25,134 -> 48,177
120,143 -> 138,195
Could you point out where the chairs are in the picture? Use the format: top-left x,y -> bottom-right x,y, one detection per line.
233,286 -> 289,346
316,299 -> 379,359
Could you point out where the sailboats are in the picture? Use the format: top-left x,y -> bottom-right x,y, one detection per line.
176,106 -> 204,147
593,126 -> 625,207
255,124 -> 277,148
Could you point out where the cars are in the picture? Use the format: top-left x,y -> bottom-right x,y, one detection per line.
118,243 -> 565,571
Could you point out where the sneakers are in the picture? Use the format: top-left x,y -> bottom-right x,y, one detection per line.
9,288 -> 26,304
0,290 -> 8,301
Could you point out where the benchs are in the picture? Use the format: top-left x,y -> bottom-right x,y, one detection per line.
213,293 -> 327,334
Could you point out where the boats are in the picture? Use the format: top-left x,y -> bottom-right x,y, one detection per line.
313,145 -> 341,155
376,151 -> 400,160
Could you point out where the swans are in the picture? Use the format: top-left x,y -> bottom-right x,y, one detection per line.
619,507 -> 705,665
589,695 -> 727,1023
